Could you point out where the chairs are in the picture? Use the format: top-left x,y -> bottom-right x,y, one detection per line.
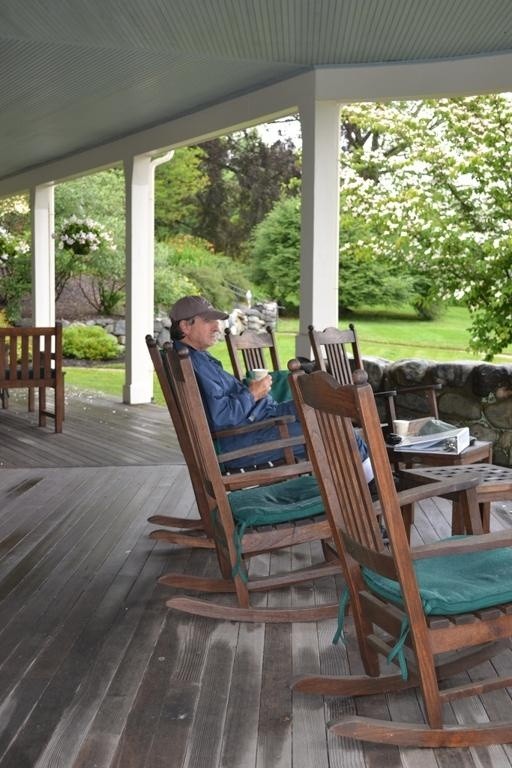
144,336 -> 378,625
222,327 -> 441,492
285,358 -> 510,750
0,324 -> 68,436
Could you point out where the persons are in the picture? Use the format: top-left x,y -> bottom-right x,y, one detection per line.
170,295 -> 397,497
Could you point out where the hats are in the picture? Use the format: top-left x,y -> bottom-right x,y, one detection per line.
169,295 -> 229,322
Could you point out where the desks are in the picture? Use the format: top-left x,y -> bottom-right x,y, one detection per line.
392,432 -> 510,537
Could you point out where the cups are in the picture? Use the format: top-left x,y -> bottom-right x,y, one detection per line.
252,368 -> 268,380
392,420 -> 409,437
